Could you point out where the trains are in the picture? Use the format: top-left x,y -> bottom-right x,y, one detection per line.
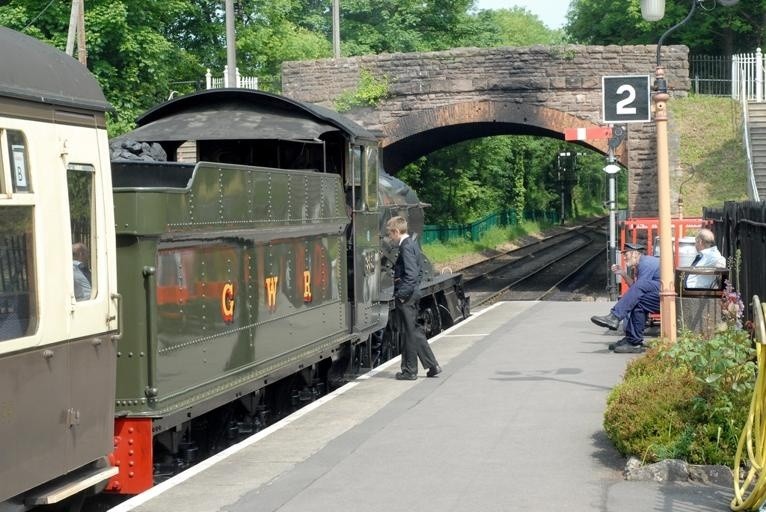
0,21 -> 472,512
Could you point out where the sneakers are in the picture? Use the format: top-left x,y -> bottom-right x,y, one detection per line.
590,312 -> 620,331
396,372 -> 418,380
609,337 -> 642,354
426,364 -> 441,377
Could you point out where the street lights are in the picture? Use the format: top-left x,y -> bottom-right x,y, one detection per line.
636,1 -> 748,350
601,144 -> 623,304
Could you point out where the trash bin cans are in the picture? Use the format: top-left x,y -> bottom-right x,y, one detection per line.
674,267 -> 729,339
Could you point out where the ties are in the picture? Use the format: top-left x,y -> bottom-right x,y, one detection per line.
683,255 -> 702,289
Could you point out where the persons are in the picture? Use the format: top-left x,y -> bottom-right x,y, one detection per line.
609,242 -> 661,351
590,228 -> 727,354
72,242 -> 91,302
386,216 -> 442,381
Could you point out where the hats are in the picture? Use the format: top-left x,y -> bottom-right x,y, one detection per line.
620,243 -> 647,254
